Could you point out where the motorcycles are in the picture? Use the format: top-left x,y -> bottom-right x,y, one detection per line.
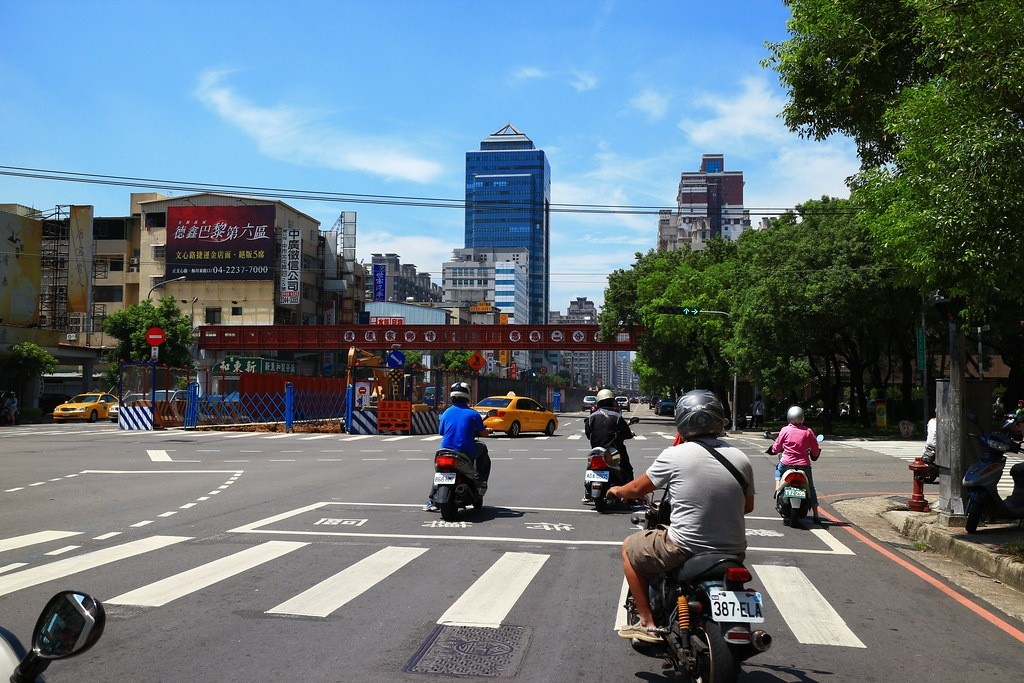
433,409 -> 495,522
603,485 -> 773,683
583,416 -> 640,513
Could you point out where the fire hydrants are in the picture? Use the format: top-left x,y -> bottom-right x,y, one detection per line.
907,457 -> 931,512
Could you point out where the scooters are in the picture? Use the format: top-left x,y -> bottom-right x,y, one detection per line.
0,590 -> 105,683
763,430 -> 825,528
956,416 -> 1024,533
997,412 -> 1024,441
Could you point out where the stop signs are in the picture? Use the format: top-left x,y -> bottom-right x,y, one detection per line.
359,387 -> 366,394
146,327 -> 165,346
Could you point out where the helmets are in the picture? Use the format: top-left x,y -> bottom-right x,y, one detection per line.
675,389 -> 723,441
595,389 -> 615,407
787,406 -> 804,424
450,382 -> 471,402
1017,400 -> 1024,408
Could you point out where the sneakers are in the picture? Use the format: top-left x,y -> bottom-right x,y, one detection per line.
478,487 -> 487,496
421,500 -> 438,511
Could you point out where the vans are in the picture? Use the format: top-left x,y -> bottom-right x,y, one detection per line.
423,386 -> 445,406
320,363 -> 346,378
615,396 -> 630,412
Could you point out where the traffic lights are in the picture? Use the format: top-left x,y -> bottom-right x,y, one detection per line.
437,364 -> 443,369
118,351 -> 125,363
935,299 -> 959,323
656,306 -> 700,316
412,363 -> 418,369
463,369 -> 468,378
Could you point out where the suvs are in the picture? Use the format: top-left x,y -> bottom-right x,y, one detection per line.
649,395 -> 662,410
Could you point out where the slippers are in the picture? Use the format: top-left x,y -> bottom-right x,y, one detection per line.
618,622 -> 664,643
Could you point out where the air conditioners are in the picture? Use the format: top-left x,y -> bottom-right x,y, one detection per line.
66,334 -> 75,340
129,258 -> 138,265
130,267 -> 137,273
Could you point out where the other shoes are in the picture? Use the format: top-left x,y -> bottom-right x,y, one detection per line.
581,496 -> 592,502
811,516 -> 821,522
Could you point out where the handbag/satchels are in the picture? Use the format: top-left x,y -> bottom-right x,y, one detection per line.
658,501 -> 671,529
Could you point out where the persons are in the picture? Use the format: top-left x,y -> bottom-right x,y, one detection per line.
606,388 -> 755,643
0,391 -> 18,427
1013,400 -> 1024,442
772,405 -> 822,524
927,408 -> 937,445
422,381 -> 491,511
581,389 -> 635,504
749,394 -> 766,432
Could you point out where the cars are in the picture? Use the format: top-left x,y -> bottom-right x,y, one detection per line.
469,391 -> 559,438
582,396 -> 597,411
168,390 -> 188,401
52,389 -> 120,423
142,390 -> 175,401
629,396 -> 651,404
796,400 -> 861,421
654,397 -> 677,416
108,393 -> 149,423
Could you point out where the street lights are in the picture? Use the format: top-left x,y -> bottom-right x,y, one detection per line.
191,297 -> 198,329
